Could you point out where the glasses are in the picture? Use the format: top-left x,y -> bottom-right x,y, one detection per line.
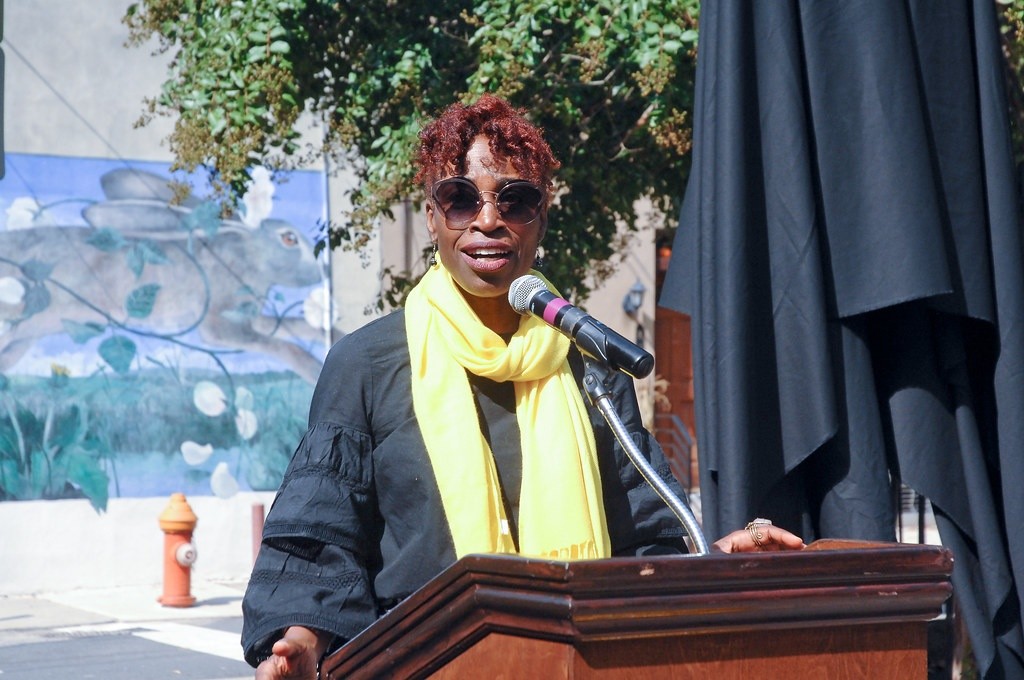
428,178 -> 549,227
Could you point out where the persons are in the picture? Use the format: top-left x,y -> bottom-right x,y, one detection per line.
240,94 -> 809,680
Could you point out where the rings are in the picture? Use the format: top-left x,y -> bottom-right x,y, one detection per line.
745,516 -> 772,546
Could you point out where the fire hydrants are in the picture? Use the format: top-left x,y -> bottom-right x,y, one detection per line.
157,492 -> 198,609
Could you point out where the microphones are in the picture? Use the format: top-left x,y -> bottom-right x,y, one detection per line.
507,275 -> 654,380
